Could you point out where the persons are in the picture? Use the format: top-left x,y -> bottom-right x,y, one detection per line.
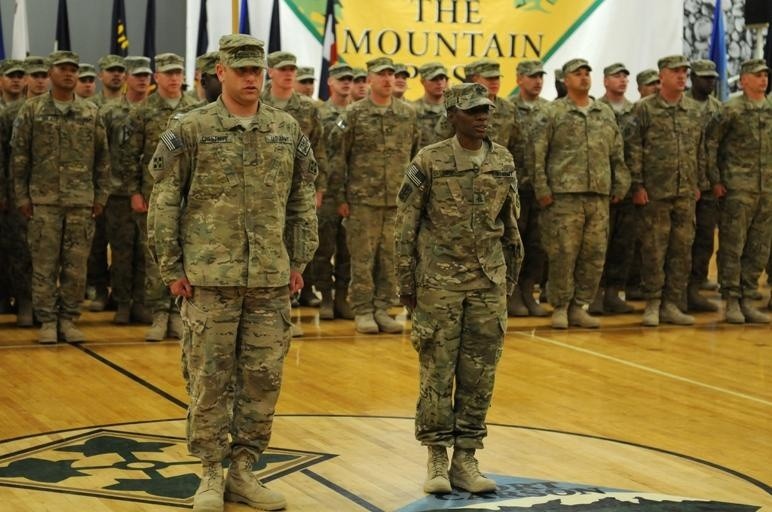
393,64 -> 416,104
506,60 -> 551,316
681,60 -> 721,312
10,52 -> 113,343
586,63 -> 637,315
527,58 -> 631,329
394,81 -> 524,495
119,53 -> 186,341
164,58 -> 241,400
317,64 -> 356,320
76,63 -> 103,298
260,50 -> 328,335
405,62 -> 457,325
83,55 -> 133,312
625,69 -> 662,300
429,60 -> 524,189
147,34 -> 319,512
329,59 -> 404,333
462,63 -> 476,86
349,71 -> 367,102
553,68 -> 567,102
624,55 -> 707,326
290,65 -> 320,308
705,59 -> 770,324
99,55 -> 155,324
0,56 -> 51,327
188,54 -> 198,102
1,59 -> 27,315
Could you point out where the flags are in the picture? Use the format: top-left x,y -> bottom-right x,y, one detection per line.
52,2 -> 78,53
264,0 -> 280,82
705,0 -> 731,105
108,2 -> 134,57
143,1 -> 160,76
195,0 -> 209,59
238,0 -> 251,35
316,0 -> 340,99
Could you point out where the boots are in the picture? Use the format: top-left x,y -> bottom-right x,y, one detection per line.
113,302 -> 132,325
131,301 -> 156,323
58,317 -> 85,342
624,289 -> 641,300
568,302 -> 602,328
550,307 -> 568,329
423,444 -> 452,495
353,315 -> 379,333
299,285 -> 321,308
603,287 -> 634,312
588,290 -> 605,315
335,289 -> 355,319
224,456 -> 286,511
523,279 -> 548,316
194,462 -> 225,512
292,325 -> 305,337
725,298 -> 745,325
144,312 -> 168,341
701,280 -> 719,290
450,448 -> 498,494
89,288 -> 109,311
509,287 -> 529,316
687,291 -> 719,313
743,289 -> 763,300
642,300 -> 660,326
740,297 -> 772,323
373,309 -> 404,333
17,298 -> 34,327
659,300 -> 696,325
319,292 -> 335,320
37,322 -> 58,344
167,312 -> 185,340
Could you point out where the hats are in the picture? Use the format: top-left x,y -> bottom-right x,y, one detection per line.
740,58 -> 769,74
392,64 -> 409,76
296,66 -> 316,82
636,69 -> 660,85
267,50 -> 297,69
48,51 -> 81,68
23,56 -> 49,74
154,53 -> 185,73
329,64 -> 355,79
442,82 -> 497,111
77,64 -> 95,78
562,59 -> 592,73
472,60 -> 505,80
690,60 -> 719,79
124,56 -> 152,75
603,62 -> 631,76
657,55 -> 691,70
198,51 -> 220,75
464,63 -> 475,74
0,59 -> 26,75
555,69 -> 564,83
218,34 -> 267,70
353,68 -> 368,81
516,62 -> 547,78
365,57 -> 398,74
419,62 -> 449,81
99,55 -> 128,70
